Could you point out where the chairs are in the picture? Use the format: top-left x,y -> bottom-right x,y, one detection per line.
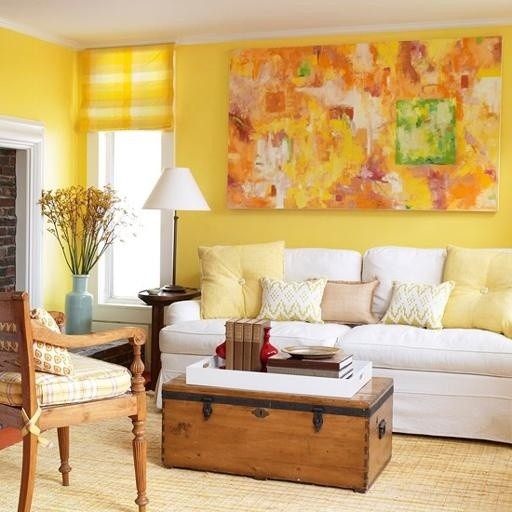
0,291 -> 148,512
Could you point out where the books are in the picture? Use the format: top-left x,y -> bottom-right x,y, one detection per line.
266,351 -> 355,380
225,317 -> 271,372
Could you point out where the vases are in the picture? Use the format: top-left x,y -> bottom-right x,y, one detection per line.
64,274 -> 93,353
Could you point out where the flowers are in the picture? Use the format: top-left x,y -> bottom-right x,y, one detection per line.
36,185 -> 137,274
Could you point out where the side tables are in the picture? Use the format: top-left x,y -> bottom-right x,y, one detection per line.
139,287 -> 200,389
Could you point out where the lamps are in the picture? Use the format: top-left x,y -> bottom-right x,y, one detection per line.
142,168 -> 210,293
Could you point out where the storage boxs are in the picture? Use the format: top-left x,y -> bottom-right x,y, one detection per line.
159,373 -> 394,493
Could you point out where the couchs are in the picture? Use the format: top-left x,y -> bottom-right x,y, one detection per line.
155,299 -> 512,445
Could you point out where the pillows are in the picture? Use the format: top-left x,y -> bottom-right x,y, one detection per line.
306,278 -> 380,324
256,277 -> 328,324
0,307 -> 72,375
283,248 -> 362,282
441,245 -> 511,339
362,247 -> 447,318
197,241 -> 284,320
378,281 -> 457,329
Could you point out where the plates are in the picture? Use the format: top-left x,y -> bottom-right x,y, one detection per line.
281,345 -> 343,359
